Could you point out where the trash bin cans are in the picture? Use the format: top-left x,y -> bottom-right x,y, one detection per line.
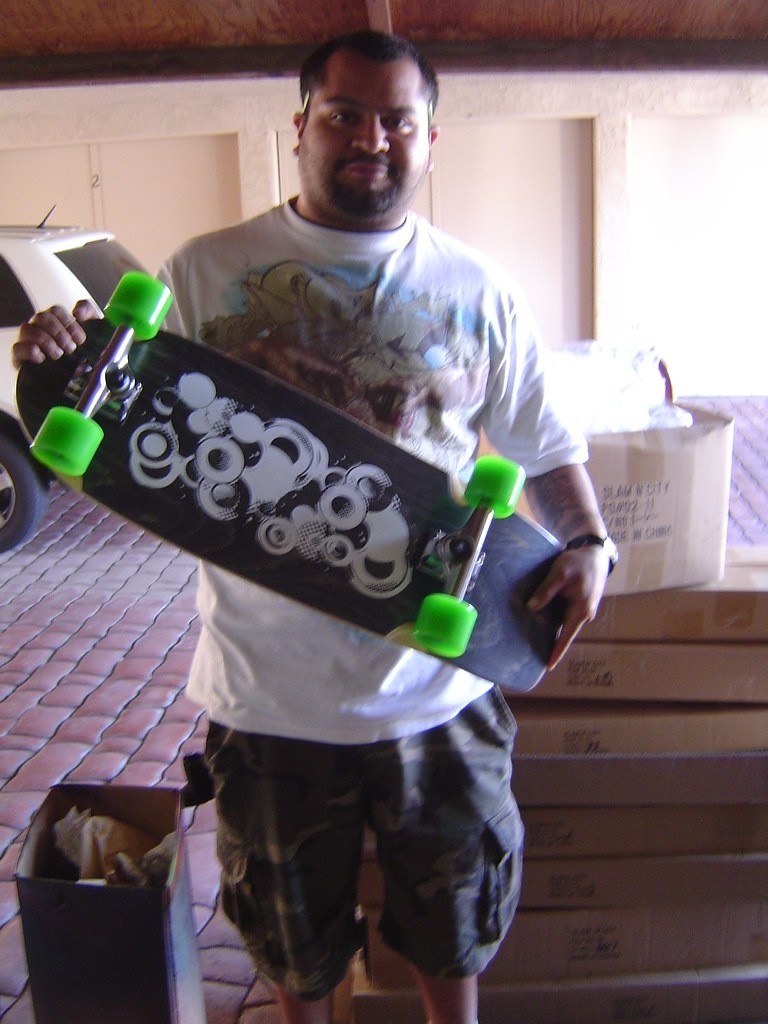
13,782 -> 207,1024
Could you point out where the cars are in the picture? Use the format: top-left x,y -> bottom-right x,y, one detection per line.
0,225 -> 154,555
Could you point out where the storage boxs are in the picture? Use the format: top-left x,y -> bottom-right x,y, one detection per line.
477,405 -> 733,598
13,784 -> 206,1024
571,546 -> 768,641
499,707 -> 768,751
360,751 -> 768,1024
509,642 -> 768,703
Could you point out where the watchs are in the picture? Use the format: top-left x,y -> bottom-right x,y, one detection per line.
564,535 -> 620,576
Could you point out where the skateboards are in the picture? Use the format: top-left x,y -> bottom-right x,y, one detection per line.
14,268 -> 569,694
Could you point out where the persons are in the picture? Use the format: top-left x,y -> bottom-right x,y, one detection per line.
11,31 -> 622,1023
